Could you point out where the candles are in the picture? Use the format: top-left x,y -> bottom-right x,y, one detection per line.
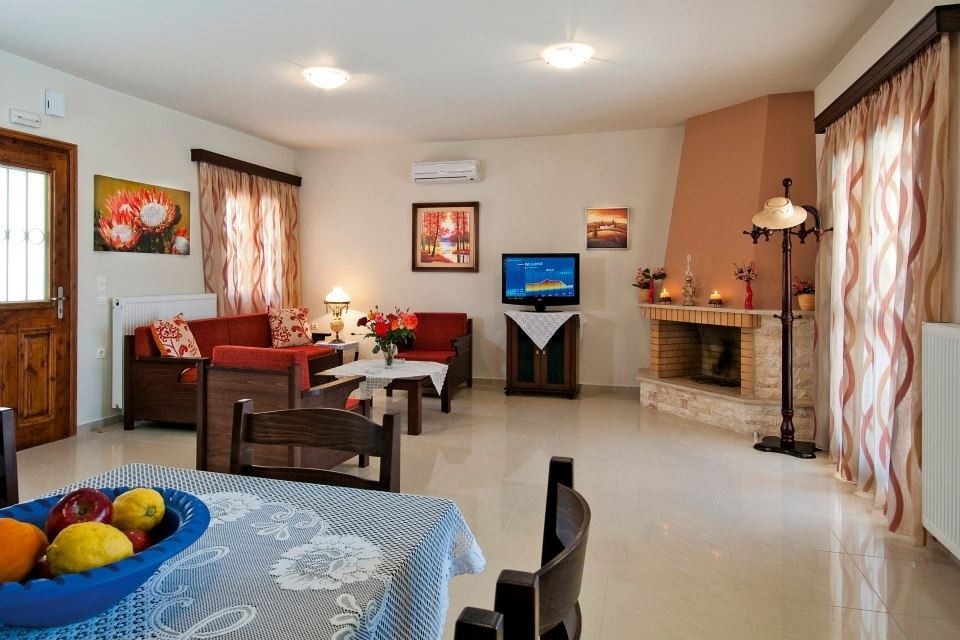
684,253 -> 693,275
707,289 -> 719,299
659,287 -> 669,297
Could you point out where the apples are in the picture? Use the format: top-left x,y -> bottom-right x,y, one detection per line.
30,487 -> 148,582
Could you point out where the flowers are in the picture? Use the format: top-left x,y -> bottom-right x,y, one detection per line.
632,266 -> 667,290
730,262 -> 758,282
793,278 -> 815,296
352,307 -> 422,352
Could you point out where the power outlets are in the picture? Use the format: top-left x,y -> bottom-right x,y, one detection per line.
310,322 -> 319,331
96,347 -> 105,360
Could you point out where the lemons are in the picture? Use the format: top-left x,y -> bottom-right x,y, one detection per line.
110,488 -> 165,531
46,521 -> 136,575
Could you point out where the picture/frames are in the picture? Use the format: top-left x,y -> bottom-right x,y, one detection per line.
584,205 -> 631,253
408,199 -> 481,274
90,172 -> 195,258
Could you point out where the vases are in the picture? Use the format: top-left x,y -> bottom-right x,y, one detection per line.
639,288 -> 649,303
796,291 -> 815,310
648,279 -> 655,303
742,280 -> 754,310
377,343 -> 405,371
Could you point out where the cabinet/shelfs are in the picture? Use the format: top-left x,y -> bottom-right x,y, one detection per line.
500,311 -> 582,401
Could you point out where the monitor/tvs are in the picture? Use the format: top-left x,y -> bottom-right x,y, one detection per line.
501,252 -> 580,313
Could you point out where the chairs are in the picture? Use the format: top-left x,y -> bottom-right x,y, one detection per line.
228,391 -> 409,494
453,456 -> 591,640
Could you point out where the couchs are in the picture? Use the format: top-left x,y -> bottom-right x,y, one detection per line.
186,348 -> 377,473
116,310 -> 342,436
392,307 -> 480,418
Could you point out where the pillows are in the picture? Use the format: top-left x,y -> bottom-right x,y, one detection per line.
149,312 -> 203,371
268,305 -> 312,350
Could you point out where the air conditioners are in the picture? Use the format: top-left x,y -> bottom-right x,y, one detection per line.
407,158 -> 478,188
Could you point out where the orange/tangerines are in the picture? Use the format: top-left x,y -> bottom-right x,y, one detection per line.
0,518 -> 48,585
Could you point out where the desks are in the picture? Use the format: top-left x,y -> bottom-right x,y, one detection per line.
0,460 -> 460,640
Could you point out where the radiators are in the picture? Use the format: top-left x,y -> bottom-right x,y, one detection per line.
919,324 -> 960,565
108,293 -> 220,413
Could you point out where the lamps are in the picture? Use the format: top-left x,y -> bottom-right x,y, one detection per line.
540,41 -> 597,71
325,287 -> 350,343
302,64 -> 351,92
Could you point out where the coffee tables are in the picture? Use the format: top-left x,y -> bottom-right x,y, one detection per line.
309,360 -> 454,445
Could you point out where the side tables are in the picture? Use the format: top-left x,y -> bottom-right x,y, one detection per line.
315,340 -> 359,362
